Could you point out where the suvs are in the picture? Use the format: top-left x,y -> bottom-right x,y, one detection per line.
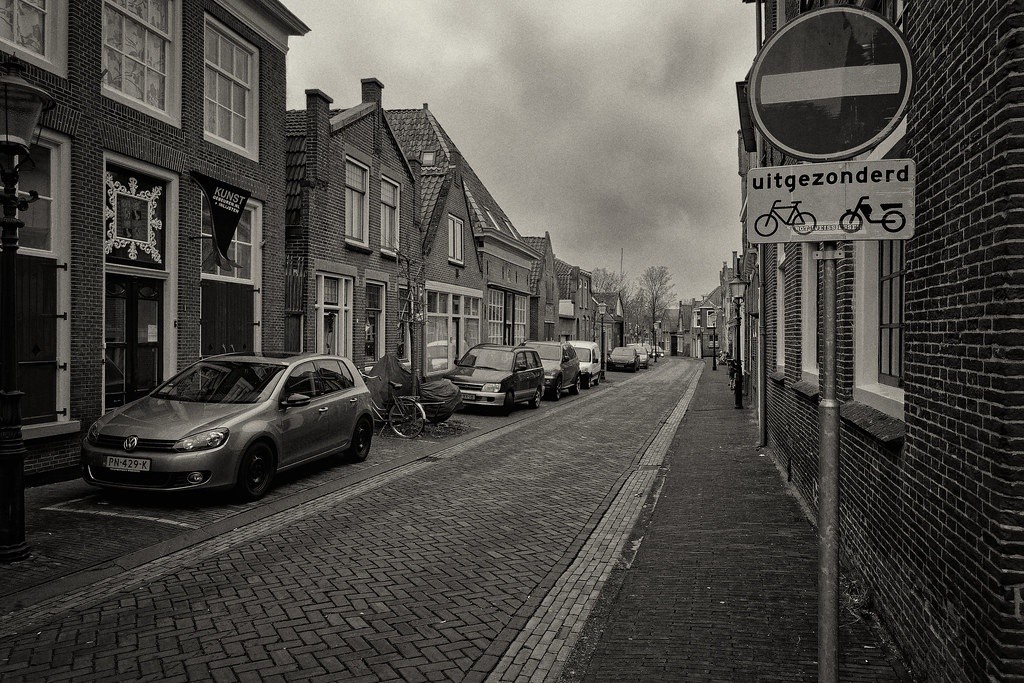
517,337 -> 582,402
440,342 -> 546,417
426,338 -> 469,371
564,340 -> 603,389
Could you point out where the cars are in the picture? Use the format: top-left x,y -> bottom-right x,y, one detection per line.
652,346 -> 665,358
626,341 -> 655,367
607,346 -> 640,373
80,349 -> 375,502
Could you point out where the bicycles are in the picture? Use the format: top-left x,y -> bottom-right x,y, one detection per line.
356,365 -> 426,439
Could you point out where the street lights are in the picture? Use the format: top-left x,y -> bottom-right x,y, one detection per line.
650,323 -> 659,362
596,300 -> 609,380
728,273 -> 751,409
710,312 -> 718,371
0,50 -> 57,557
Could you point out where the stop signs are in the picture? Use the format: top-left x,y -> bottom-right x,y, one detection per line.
747,2 -> 917,162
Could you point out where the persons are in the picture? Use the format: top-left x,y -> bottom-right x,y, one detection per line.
717,350 -> 730,365
